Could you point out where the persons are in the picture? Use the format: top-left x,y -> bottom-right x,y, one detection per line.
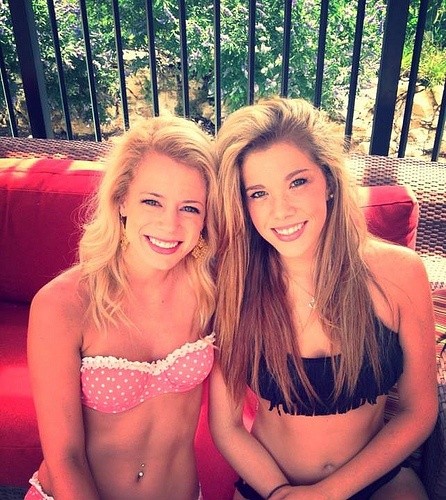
210,95 -> 438,500
24,116 -> 215,500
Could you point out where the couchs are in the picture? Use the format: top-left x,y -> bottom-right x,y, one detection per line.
0,158 -> 419,500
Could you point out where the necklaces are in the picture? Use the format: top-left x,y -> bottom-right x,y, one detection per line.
289,273 -> 316,308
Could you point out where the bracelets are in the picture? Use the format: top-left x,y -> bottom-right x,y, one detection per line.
265,483 -> 291,499
